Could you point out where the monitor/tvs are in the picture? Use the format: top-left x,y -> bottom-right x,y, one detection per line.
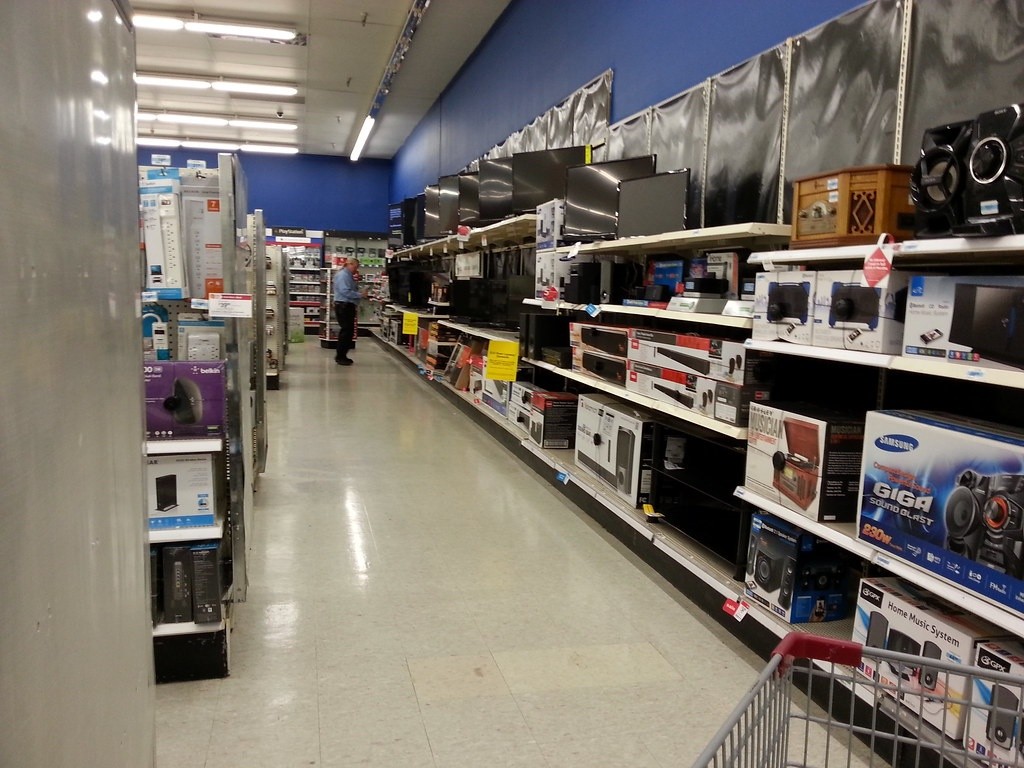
948,284 -> 1024,371
387,144 -> 691,332
886,628 -> 921,681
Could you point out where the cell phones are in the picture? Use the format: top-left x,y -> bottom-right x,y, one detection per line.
847,329 -> 863,343
786,324 -> 796,335
920,329 -> 943,344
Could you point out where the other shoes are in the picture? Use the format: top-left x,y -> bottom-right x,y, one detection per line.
338,358 -> 353,364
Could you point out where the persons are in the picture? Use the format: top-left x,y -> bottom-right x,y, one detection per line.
333,257 -> 368,365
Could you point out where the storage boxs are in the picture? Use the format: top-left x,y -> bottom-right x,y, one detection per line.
289,306 -> 305,344
142,359 -> 224,439
430,198 -> 1024,766
145,452 -> 219,527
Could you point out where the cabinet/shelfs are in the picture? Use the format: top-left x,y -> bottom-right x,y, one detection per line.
520,216 -> 793,606
138,154 -> 330,638
732,214 -> 1024,768
367,211 -> 538,440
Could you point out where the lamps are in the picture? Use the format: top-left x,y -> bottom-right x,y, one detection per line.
131,10 -> 376,162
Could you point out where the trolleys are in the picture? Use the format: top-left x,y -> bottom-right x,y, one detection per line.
694,631 -> 1024,768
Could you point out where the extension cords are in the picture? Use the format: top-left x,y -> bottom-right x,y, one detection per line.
141,193 -> 224,363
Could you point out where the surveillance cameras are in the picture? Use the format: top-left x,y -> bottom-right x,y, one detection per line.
277,112 -> 284,118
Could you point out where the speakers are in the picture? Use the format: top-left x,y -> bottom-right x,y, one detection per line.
518,313 -> 577,361
615,426 -> 635,494
920,641 -> 942,690
747,535 -> 796,611
985,684 -> 1019,750
866,612 -> 889,651
